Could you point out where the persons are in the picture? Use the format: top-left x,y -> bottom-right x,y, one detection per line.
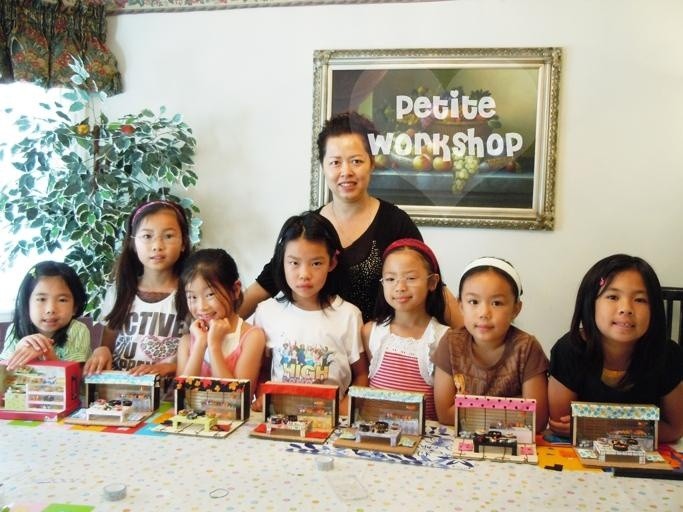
231,113 -> 463,329
82,201 -> 188,379
250,213 -> 369,415
428,256 -> 547,434
174,248 -> 265,406
360,238 -> 453,422
0,260 -> 90,372
547,253 -> 682,445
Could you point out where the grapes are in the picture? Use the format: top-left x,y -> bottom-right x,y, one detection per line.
452,154 -> 479,193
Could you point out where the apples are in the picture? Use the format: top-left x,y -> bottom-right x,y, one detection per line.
413,145 -> 452,172
375,155 -> 388,168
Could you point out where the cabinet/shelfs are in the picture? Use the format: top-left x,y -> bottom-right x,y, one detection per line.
1,372 -> 64,411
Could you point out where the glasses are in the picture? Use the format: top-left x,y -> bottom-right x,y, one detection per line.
132,232 -> 181,245
379,274 -> 427,289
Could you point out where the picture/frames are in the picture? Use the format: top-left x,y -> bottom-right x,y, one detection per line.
305,46 -> 563,231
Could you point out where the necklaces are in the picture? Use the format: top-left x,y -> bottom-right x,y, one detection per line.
329,196 -> 372,245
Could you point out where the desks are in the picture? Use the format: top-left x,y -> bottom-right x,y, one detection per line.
0,411 -> 681,512
83,401 -> 645,467
